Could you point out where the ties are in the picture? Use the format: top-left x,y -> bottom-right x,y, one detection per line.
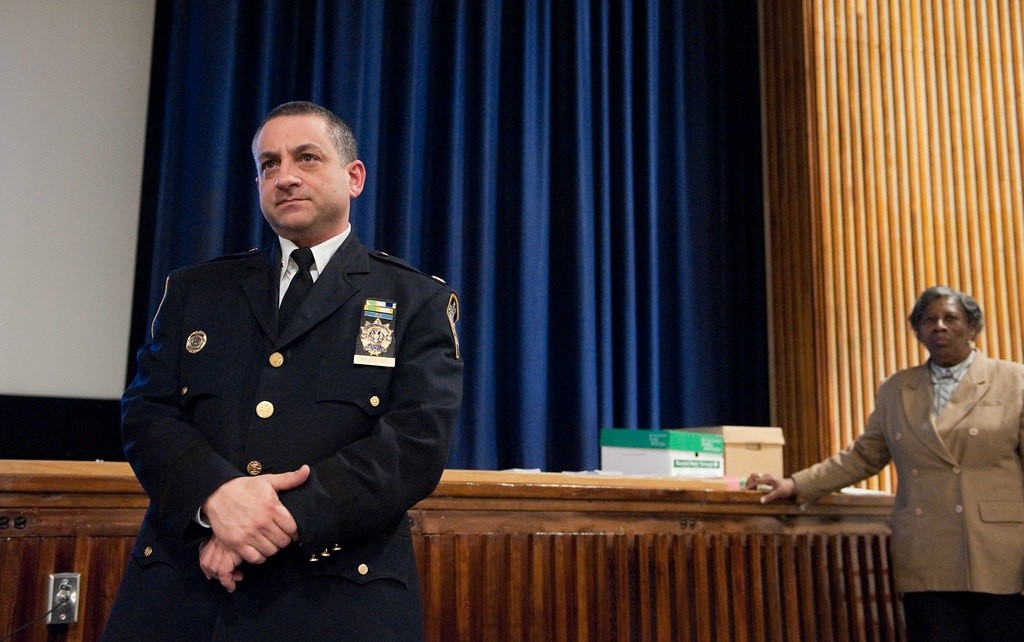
279,247 -> 315,336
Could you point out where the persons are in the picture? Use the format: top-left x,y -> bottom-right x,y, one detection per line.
743,284 -> 1024,642
97,99 -> 466,642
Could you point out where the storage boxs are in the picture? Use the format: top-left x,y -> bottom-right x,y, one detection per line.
671,424 -> 785,483
599,424 -> 725,479
